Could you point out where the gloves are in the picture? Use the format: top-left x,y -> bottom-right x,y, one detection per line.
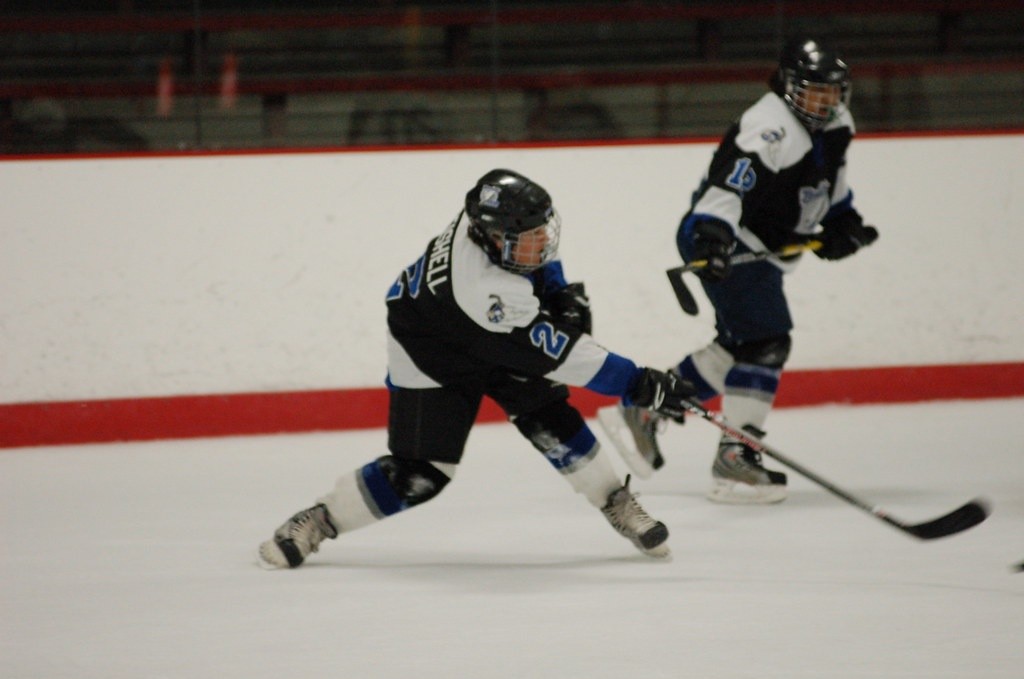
626,364 -> 695,424
546,280 -> 592,334
690,230 -> 730,279
810,224 -> 864,261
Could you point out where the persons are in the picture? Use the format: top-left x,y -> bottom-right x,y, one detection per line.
596,33 -> 864,503
257,168 -> 694,570
430,23 -> 477,67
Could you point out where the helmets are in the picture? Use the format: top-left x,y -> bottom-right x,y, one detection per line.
779,37 -> 849,128
464,169 -> 559,273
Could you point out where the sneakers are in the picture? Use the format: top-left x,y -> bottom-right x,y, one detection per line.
707,422 -> 789,505
600,473 -> 669,556
596,401 -> 664,478
258,503 -> 339,568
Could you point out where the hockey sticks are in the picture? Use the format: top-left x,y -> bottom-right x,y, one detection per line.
665,239 -> 823,318
680,396 -> 995,543
